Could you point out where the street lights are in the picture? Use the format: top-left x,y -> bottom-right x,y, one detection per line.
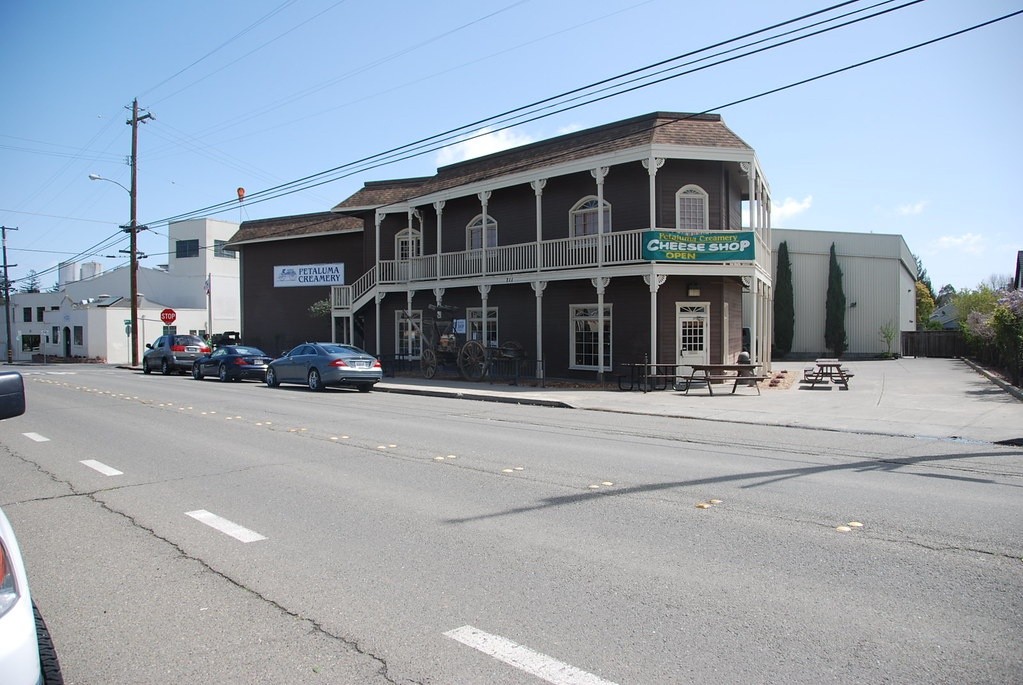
88,173 -> 138,367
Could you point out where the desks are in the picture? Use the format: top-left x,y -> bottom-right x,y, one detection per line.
624,364 -> 762,396
810,358 -> 848,390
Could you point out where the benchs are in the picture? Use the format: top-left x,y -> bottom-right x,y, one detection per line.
804,367 -> 855,390
615,373 -> 770,396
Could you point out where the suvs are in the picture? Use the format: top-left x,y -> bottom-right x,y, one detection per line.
143,334 -> 211,375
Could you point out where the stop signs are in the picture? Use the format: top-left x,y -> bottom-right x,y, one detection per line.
161,309 -> 176,325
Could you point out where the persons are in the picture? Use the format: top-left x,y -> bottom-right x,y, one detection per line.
737,347 -> 749,360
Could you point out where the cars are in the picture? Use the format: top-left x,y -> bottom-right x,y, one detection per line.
265,342 -> 383,392
0,372 -> 63,685
192,345 -> 276,382
